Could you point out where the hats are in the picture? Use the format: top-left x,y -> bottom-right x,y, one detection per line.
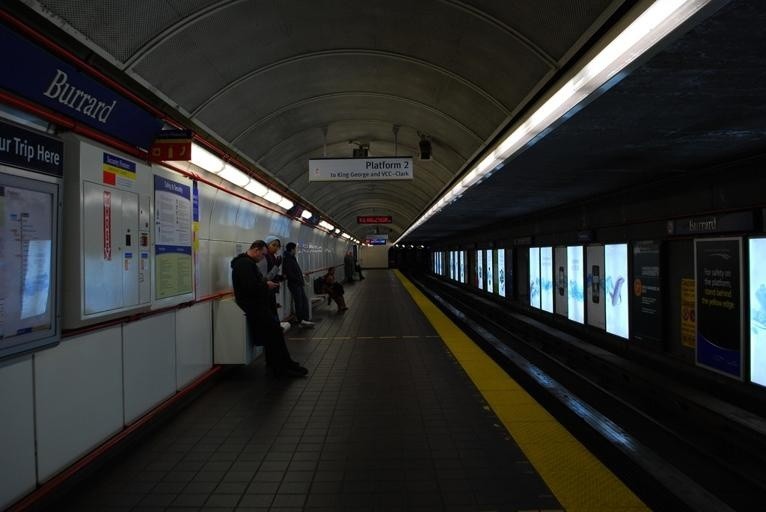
265,236 -> 280,248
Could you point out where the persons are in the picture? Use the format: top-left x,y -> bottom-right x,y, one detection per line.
324,266 -> 348,311
344,251 -> 366,282
282,242 -> 315,326
231,240 -> 308,376
255,235 -> 286,293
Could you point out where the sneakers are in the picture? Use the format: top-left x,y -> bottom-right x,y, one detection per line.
298,320 -> 315,328
